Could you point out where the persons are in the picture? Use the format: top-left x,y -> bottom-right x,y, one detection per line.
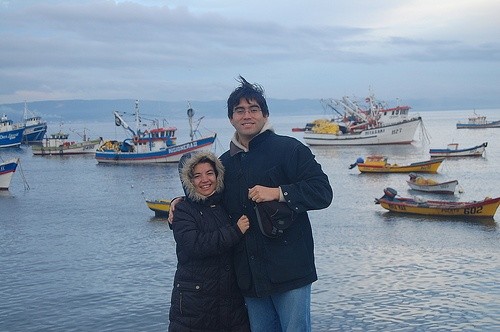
167,76 -> 333,332
167,150 -> 249,332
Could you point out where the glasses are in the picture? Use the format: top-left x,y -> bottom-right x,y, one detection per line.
232,108 -> 263,114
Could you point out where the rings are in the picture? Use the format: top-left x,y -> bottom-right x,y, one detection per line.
253,197 -> 256,201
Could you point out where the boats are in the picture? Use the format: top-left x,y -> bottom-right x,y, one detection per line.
428,141 -> 488,157
291,85 -> 422,147
347,153 -> 448,174
405,169 -> 461,197
0,149 -> 19,190
456,107 -> 500,129
144,198 -> 171,218
31,121 -> 104,156
94,98 -> 219,164
0,98 -> 48,148
374,187 -> 500,220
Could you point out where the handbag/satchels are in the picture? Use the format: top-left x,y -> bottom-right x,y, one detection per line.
255,200 -> 294,238
